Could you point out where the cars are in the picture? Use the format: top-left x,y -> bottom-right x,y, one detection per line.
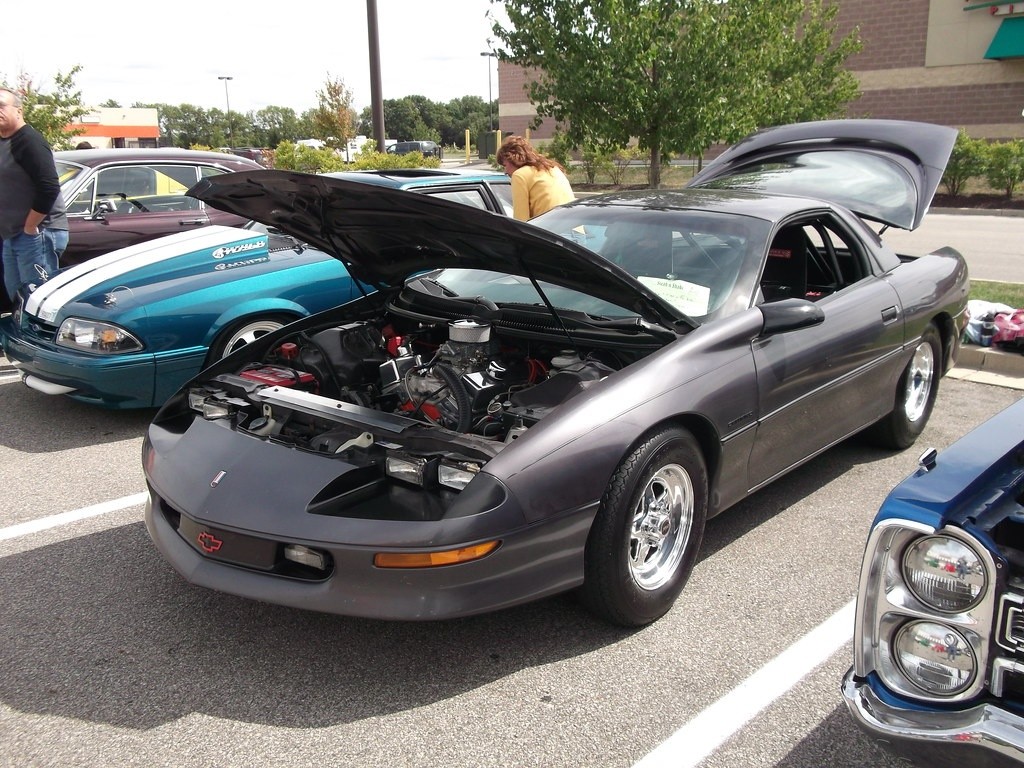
57,166 -> 190,205
31,148 -> 270,271
839,400 -> 1024,768
2,168 -> 610,407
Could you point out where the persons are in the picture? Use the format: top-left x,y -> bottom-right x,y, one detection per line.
76,141 -> 93,150
495,135 -> 588,248
0,87 -> 70,302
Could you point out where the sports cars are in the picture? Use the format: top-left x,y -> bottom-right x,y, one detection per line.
134,118 -> 970,629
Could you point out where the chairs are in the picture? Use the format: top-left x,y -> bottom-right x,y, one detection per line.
613,226 -> 673,270
726,228 -> 808,301
139,205 -> 173,213
117,202 -> 136,214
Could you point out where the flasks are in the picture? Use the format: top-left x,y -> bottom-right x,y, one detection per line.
980,313 -> 995,347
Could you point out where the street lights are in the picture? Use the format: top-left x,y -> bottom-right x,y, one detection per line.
218,76 -> 235,149
481,51 -> 497,131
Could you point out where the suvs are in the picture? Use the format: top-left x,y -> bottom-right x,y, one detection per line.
230,148 -> 265,167
385,141 -> 443,162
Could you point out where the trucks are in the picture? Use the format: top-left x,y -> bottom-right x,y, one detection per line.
294,134 -> 397,165
